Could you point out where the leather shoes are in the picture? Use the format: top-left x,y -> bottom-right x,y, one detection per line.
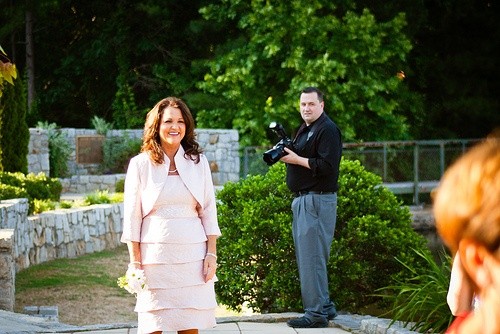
328,313 -> 337,319
287,316 -> 328,328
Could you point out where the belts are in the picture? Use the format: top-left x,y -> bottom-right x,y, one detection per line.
297,190 -> 333,196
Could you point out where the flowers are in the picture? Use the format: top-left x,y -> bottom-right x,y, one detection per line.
117,269 -> 147,296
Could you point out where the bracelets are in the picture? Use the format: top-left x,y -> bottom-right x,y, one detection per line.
206,253 -> 218,260
129,262 -> 142,265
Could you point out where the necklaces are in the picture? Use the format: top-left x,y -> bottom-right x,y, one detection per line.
168,169 -> 178,174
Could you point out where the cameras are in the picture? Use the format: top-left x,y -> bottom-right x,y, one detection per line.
263,121 -> 294,166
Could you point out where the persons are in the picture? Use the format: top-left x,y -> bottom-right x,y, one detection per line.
119,96 -> 219,334
433,131 -> 500,334
273,87 -> 342,328
